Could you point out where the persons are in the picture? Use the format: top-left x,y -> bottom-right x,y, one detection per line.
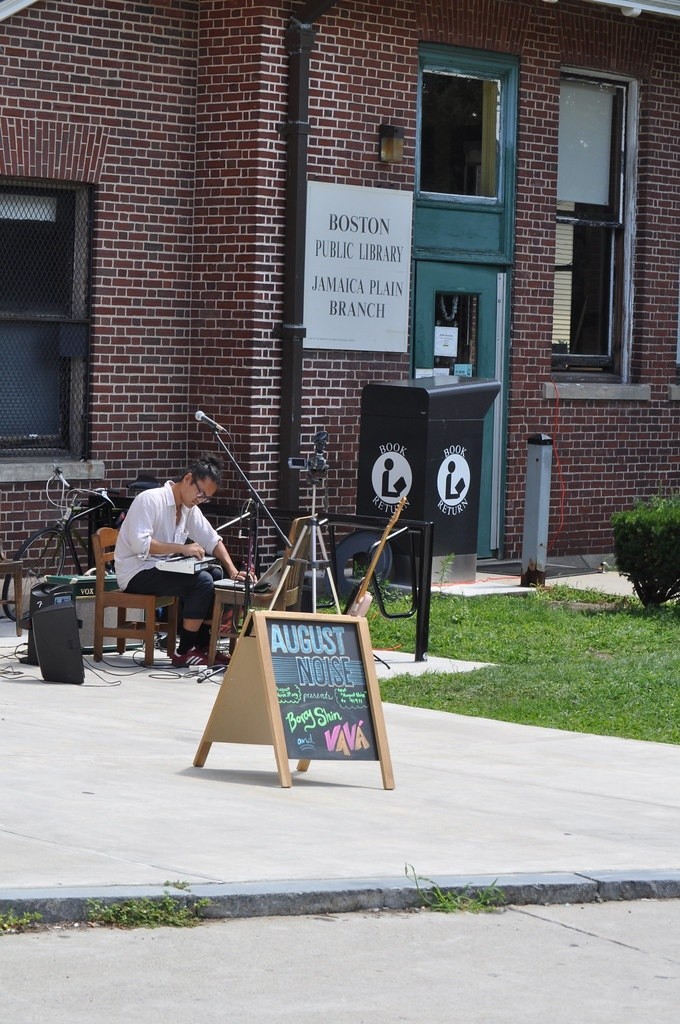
113,455 -> 257,667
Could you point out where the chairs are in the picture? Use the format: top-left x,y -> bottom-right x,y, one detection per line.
92,527 -> 177,666
208,513 -> 317,665
0,561 -> 23,636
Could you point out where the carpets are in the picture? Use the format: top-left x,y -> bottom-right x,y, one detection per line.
476,562 -> 601,579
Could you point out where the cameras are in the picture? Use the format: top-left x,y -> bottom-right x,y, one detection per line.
288,432 -> 329,471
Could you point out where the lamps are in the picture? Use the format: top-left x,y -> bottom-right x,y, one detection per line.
380,124 -> 405,164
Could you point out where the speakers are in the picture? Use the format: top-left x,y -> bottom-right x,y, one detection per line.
28,582 -> 76,665
45,575 -> 144,652
32,600 -> 85,685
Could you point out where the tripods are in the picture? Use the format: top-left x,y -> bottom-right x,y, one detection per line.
267,471 -> 342,615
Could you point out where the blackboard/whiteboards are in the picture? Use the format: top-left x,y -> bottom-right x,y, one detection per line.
204,611 -> 391,761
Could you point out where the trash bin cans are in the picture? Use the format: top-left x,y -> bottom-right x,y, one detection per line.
352,374 -> 501,587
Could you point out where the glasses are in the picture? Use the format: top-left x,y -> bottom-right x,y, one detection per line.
193,480 -> 212,504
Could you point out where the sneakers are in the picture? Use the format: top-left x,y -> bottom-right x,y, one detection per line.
202,648 -> 231,664
171,646 -> 208,667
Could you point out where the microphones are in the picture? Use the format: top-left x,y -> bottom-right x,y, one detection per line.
195,411 -> 228,434
